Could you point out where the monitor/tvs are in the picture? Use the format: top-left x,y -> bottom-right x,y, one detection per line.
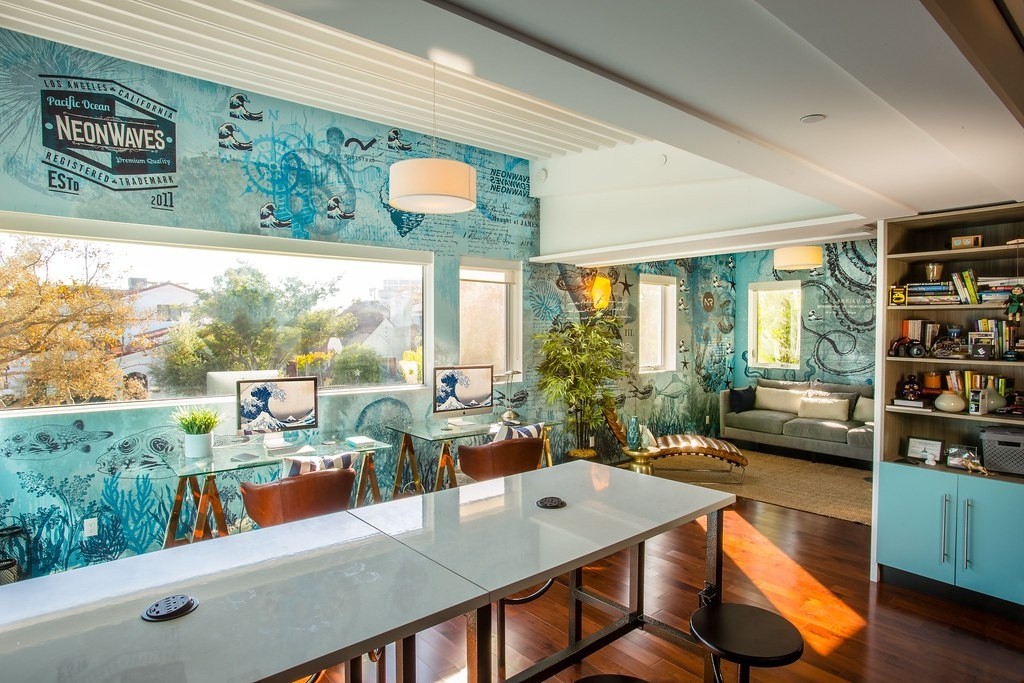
432,365 -> 494,426
237,376 -> 318,451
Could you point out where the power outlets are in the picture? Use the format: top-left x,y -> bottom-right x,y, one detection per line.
705,416 -> 711,424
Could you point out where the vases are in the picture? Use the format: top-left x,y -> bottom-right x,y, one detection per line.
186,432 -> 212,457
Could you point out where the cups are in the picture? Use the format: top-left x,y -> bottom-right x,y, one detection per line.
925,263 -> 944,283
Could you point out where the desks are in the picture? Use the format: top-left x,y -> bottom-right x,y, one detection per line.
624,443 -> 661,476
385,420 -> 561,502
0,510 -> 489,683
346,458 -> 736,683
153,435 -> 392,548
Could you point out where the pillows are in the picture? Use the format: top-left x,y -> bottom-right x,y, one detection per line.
495,422 -> 546,443
869,203 -> 1024,608
803,389 -> 858,419
282,451 -> 362,477
726,385 -> 754,414
753,385 -> 805,413
798,397 -> 849,421
851,396 -> 873,424
640,423 -> 657,447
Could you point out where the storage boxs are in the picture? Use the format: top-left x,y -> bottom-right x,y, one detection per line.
981,429 -> 1024,478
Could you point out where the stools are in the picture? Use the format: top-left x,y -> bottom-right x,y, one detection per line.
689,602 -> 803,683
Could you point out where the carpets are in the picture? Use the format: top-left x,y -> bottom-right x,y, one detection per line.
611,448 -> 873,526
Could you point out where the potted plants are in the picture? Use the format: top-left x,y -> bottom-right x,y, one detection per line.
532,324 -> 622,461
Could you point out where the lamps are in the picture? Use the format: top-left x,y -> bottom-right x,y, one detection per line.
497,370 -> 522,421
772,245 -> 824,270
388,62 -> 477,215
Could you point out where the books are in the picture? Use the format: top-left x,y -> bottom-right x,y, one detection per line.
902,318 -> 937,352
973,317 -> 1024,359
904,269 -> 1024,304
944,368 -> 1015,404
885,398 -> 934,412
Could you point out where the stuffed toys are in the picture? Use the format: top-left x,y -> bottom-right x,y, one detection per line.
1002,284 -> 1024,327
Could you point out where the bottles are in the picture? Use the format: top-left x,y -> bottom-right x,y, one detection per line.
901,375 -> 922,401
627,416 -> 640,451
640,426 -> 650,449
981,376 -> 1007,412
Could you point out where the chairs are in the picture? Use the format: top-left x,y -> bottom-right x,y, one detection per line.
603,407 -> 749,484
458,438 -> 543,489
238,470 -> 355,527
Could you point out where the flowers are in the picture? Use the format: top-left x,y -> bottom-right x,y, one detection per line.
172,405 -> 221,434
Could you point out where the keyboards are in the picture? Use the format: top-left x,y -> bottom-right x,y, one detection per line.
268,444 -> 316,456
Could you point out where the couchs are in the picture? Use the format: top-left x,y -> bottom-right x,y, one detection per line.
719,378 -> 874,470
